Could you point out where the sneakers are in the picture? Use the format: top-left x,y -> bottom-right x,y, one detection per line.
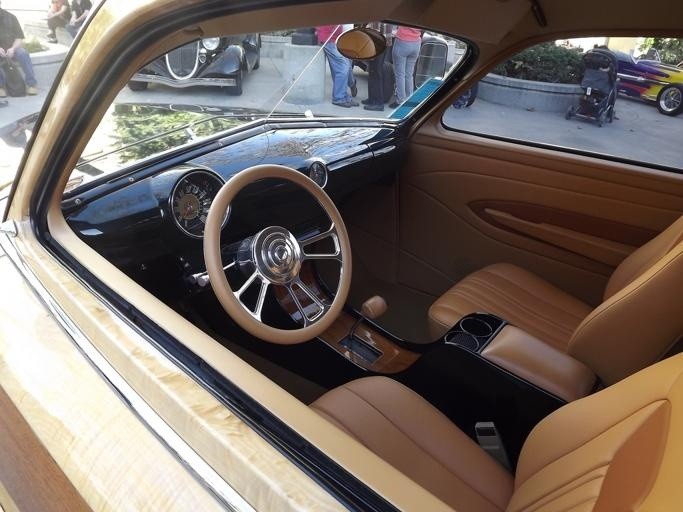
1,89 -> 7,97
351,80 -> 357,97
28,85 -> 37,95
47,29 -> 57,43
333,97 -> 401,111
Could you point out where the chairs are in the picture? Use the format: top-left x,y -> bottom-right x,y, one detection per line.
428,210 -> 683,388
306,348 -> 683,512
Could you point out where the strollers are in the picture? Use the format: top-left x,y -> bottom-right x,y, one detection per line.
565,48 -> 618,127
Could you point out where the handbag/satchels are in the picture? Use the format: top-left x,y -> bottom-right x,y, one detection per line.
3,64 -> 25,97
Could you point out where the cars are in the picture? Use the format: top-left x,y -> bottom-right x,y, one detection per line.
612,50 -> 683,116
0,0 -> 683,512
351,21 -> 479,109
128,33 -> 262,96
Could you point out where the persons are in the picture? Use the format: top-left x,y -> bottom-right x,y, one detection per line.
387,21 -> 421,109
360,20 -> 394,112
65,0 -> 92,39
40,0 -> 71,42
0,0 -> 38,97
347,57 -> 358,98
316,22 -> 361,108
